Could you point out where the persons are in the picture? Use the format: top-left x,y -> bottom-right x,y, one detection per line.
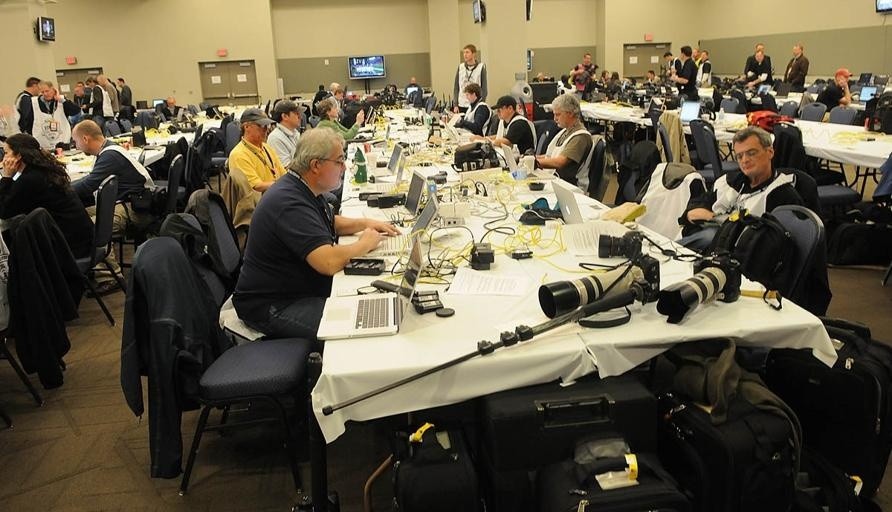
0,42 -> 892,477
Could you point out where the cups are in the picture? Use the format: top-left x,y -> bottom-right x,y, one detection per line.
526,182 -> 545,191
701,114 -> 710,121
46,147 -> 63,162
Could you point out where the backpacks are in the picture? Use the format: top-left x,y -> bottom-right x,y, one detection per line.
701,213 -> 799,294
390,416 -> 481,512
747,110 -> 792,133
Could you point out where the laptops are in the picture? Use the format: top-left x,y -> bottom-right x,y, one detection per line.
501,143 -> 557,183
417,107 -> 476,143
360,168 -> 427,224
550,180 -> 611,225
317,233 -> 424,340
407,87 -> 418,96
348,105 -> 407,195
851,85 -> 878,104
678,102 -> 700,125
753,85 -> 771,97
153,99 -> 163,108
362,194 -> 440,255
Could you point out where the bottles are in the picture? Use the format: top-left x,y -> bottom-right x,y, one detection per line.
512,144 -> 520,154
516,158 -> 527,181
353,143 -> 377,183
524,153 -> 534,172
718,108 -> 725,120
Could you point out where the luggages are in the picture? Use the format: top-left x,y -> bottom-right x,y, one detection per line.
482,375 -> 660,508
539,441 -> 688,512
775,314 -> 891,499
660,379 -> 799,509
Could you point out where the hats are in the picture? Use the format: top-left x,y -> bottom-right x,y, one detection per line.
272,99 -> 307,119
489,95 -> 517,110
833,69 -> 856,79
240,105 -> 278,127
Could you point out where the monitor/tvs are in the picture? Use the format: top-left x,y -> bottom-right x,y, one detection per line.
347,55 -> 386,79
39,16 -> 55,42
876,0 -> 892,12
473,0 -> 481,24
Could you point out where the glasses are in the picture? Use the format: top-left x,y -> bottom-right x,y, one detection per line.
315,156 -> 347,168
733,146 -> 766,162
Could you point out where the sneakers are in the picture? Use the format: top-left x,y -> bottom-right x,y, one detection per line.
86,278 -> 124,299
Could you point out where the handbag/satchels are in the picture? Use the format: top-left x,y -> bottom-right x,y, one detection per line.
825,196 -> 892,266
453,137 -> 498,170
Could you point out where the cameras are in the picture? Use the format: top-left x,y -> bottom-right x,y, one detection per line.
599,232 -> 643,258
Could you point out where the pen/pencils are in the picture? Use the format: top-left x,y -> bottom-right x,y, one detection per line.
79,172 -> 87,173
354,232 -> 389,236
446,115 -> 447,124
